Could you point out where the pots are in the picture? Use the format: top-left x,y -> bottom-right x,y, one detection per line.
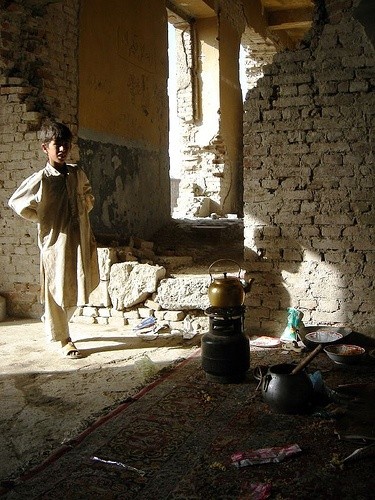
296,326 -> 353,351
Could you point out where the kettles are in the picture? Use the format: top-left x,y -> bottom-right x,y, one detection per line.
208,259 -> 255,307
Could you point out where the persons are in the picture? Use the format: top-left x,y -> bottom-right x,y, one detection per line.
8,120 -> 100,360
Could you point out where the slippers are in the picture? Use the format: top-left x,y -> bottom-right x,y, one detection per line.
57,341 -> 81,359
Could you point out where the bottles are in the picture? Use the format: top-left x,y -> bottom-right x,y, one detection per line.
260,363 -> 312,412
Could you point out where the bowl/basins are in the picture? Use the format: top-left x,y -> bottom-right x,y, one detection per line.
304,331 -> 342,342
324,344 -> 366,365
